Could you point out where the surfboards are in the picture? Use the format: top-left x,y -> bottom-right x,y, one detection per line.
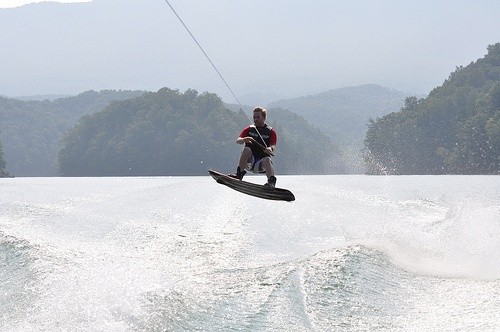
206,169 -> 295,202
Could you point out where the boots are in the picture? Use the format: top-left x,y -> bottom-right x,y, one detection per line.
227,166 -> 247,180
264,176 -> 276,188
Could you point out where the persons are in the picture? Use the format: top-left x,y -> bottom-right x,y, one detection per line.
227,106 -> 276,187
